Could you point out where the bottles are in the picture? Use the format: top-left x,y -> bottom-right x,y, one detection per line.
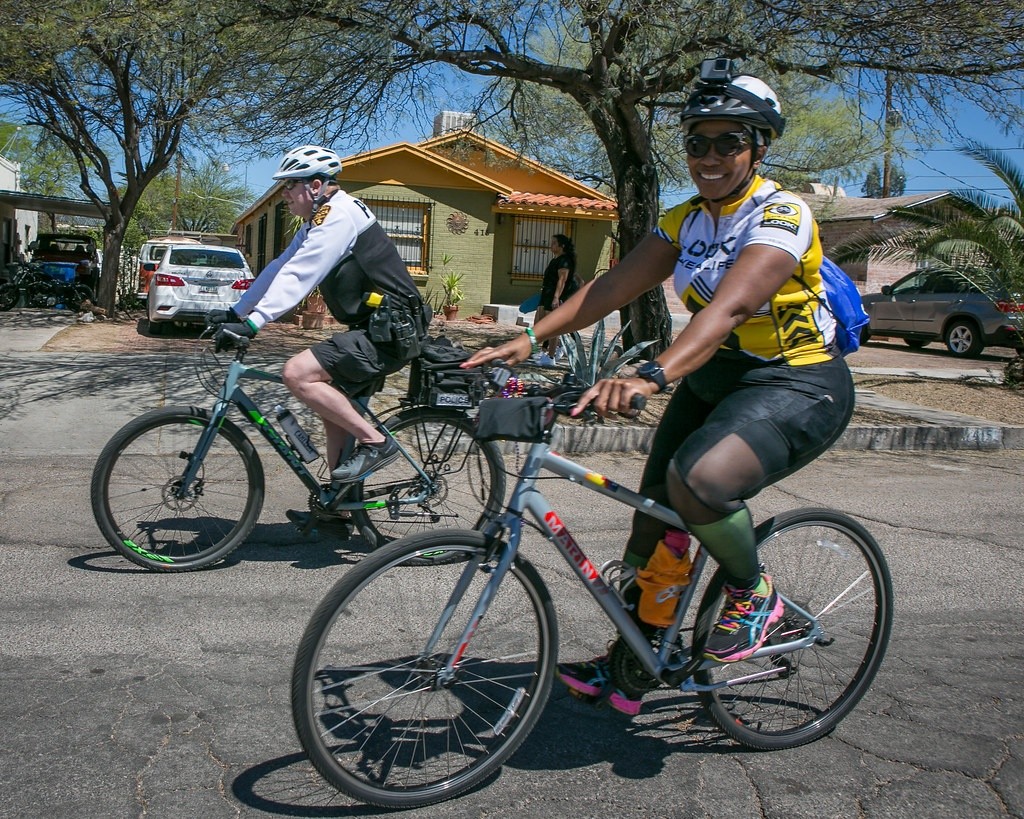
274,403 -> 320,464
636,531 -> 691,628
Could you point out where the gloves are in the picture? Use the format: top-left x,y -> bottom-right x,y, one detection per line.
203,308 -> 238,332
212,322 -> 257,353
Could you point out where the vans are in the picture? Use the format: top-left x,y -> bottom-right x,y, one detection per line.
138,237 -> 204,295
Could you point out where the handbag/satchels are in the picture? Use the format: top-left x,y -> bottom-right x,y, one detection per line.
409,340 -> 492,409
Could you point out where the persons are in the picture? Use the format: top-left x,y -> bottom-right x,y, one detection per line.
206,145 -> 432,541
532,234 -> 577,365
459,73 -> 856,714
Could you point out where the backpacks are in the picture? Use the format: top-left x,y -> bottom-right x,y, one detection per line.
764,190 -> 873,357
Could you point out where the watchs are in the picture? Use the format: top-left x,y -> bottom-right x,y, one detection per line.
637,361 -> 667,393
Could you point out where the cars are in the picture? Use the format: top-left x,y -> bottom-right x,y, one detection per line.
143,244 -> 259,334
859,265 -> 1024,359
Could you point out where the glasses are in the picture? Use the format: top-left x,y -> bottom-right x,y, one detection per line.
682,132 -> 748,158
283,179 -> 312,191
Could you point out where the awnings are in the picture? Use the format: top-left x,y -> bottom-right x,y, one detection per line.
0,189 -> 112,218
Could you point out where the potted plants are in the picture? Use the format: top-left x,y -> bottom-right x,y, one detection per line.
442,255 -> 464,321
305,288 -> 327,313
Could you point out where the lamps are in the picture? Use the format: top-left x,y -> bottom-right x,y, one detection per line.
497,214 -> 504,225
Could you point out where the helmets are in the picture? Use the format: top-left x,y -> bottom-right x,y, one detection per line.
270,145 -> 343,180
679,71 -> 779,139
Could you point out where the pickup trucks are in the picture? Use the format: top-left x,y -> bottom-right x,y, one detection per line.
30,231 -> 100,295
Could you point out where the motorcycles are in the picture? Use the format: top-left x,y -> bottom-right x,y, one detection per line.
0,251 -> 93,317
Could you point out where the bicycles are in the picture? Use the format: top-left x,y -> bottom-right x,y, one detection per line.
90,316 -> 511,573
292,347 -> 895,810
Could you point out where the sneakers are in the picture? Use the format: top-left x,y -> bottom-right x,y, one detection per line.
536,355 -> 556,367
555,348 -> 564,359
703,564 -> 785,662
555,640 -> 646,715
285,505 -> 354,541
532,350 -> 545,360
331,434 -> 400,483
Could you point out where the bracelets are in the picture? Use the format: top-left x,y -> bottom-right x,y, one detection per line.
524,328 -> 537,356
